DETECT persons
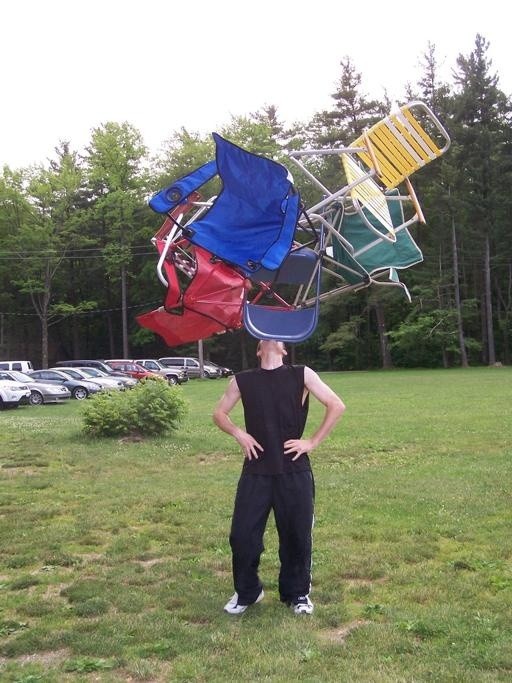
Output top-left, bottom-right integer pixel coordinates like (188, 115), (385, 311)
(211, 334), (346, 618)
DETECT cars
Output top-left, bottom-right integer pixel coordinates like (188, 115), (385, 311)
(0, 356), (232, 411)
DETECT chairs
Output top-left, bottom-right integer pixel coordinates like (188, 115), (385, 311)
(151, 133), (299, 275)
(287, 101), (451, 242)
(138, 191), (246, 348)
(301, 187), (429, 312)
(241, 210), (330, 343)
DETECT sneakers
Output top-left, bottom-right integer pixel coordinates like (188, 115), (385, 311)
(224, 589), (266, 615)
(292, 594), (313, 615)
